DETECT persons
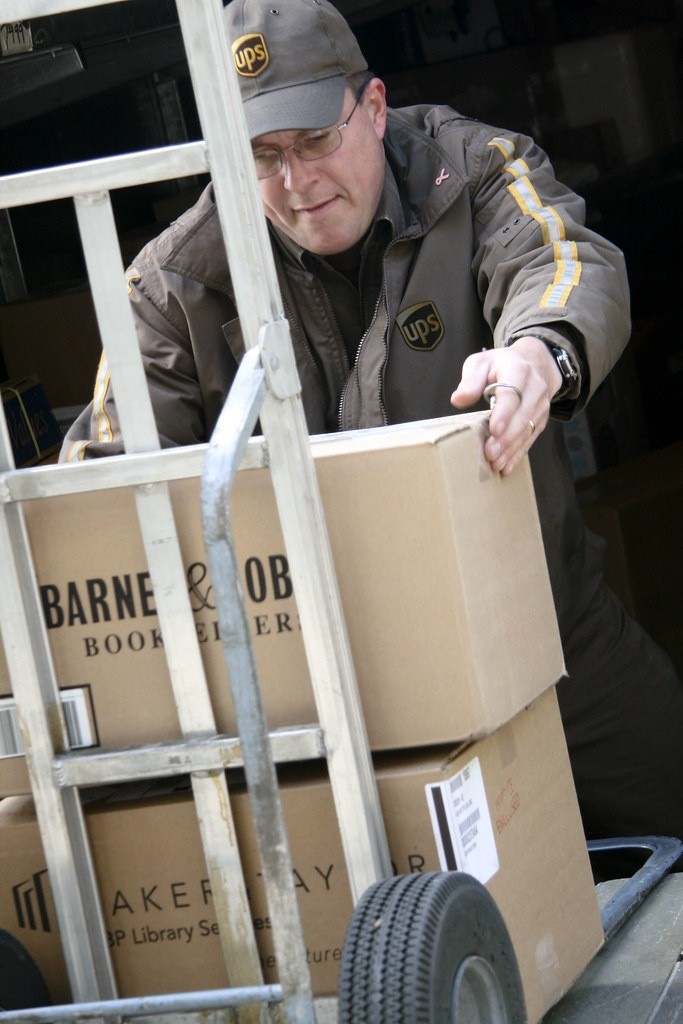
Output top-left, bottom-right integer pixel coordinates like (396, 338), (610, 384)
(60, 0), (683, 844)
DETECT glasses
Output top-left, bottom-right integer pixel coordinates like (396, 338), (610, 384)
(252, 92), (371, 180)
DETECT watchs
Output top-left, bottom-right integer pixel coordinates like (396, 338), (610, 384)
(553, 344), (578, 397)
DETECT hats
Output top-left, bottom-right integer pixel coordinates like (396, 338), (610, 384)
(225, 0), (369, 139)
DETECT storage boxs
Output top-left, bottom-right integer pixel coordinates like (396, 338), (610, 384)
(0, 24), (683, 1024)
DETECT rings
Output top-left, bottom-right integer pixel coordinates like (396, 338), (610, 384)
(530, 420), (536, 430)
